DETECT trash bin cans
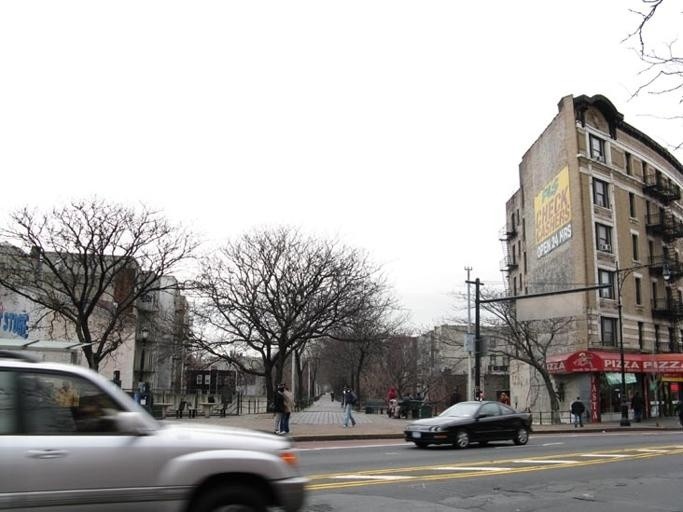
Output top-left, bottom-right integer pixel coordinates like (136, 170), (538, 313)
(410, 400), (433, 419)
(134, 382), (153, 415)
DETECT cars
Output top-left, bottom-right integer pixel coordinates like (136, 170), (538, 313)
(1, 357), (309, 512)
(403, 400), (533, 449)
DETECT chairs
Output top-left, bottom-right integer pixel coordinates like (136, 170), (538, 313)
(170, 399), (200, 418)
(215, 401), (227, 418)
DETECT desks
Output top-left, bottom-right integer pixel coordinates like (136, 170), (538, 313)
(152, 402), (172, 418)
(199, 402), (216, 418)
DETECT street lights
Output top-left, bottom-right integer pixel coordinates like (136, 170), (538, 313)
(138, 327), (150, 382)
(615, 260), (671, 426)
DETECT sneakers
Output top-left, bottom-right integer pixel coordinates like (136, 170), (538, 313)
(275, 428), (286, 434)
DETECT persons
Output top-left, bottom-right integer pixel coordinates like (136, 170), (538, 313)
(630, 391), (644, 422)
(571, 396), (585, 427)
(342, 384), (358, 428)
(499, 392), (510, 406)
(278, 383), (292, 434)
(53, 380), (79, 407)
(331, 391), (335, 401)
(272, 384), (284, 434)
(385, 387), (422, 418)
